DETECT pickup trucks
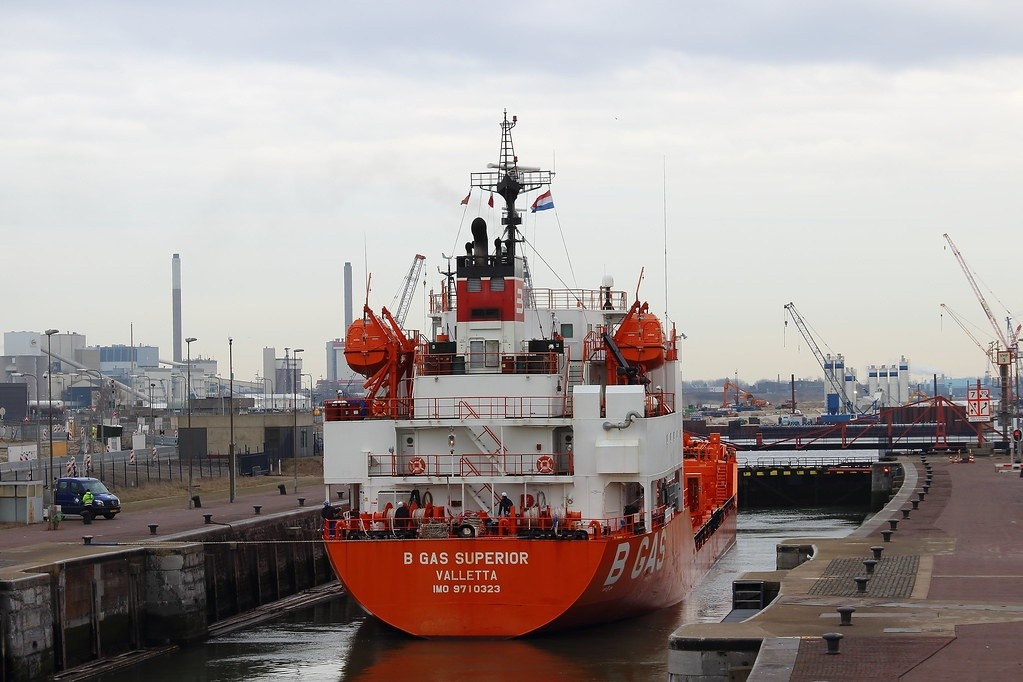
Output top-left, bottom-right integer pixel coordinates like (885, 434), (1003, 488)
(55, 477), (121, 521)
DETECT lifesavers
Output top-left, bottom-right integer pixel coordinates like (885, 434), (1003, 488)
(457, 524), (475, 538)
(537, 456), (553, 473)
(409, 457), (425, 474)
(372, 401), (388, 418)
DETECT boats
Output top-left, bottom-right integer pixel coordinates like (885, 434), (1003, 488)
(613, 313), (665, 372)
(343, 317), (401, 378)
(319, 108), (738, 639)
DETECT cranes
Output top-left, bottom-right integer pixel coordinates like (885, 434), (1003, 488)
(939, 233), (1023, 442)
(783, 302), (880, 424)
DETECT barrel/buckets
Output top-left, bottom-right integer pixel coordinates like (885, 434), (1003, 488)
(516, 355), (557, 373)
(501, 356), (514, 373)
(425, 356), (466, 375)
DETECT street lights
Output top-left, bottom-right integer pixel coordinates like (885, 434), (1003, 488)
(10, 372), (41, 473)
(68, 373), (93, 473)
(51, 375), (67, 422)
(151, 383), (156, 418)
(77, 368), (105, 482)
(294, 349), (305, 495)
(129, 375), (154, 449)
(45, 328), (60, 530)
(265, 379), (274, 414)
(207, 376), (220, 415)
(185, 337), (198, 510)
(227, 337), (235, 504)
(299, 373), (312, 409)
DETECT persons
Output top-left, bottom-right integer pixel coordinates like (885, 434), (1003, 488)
(394, 502), (410, 538)
(321, 500), (342, 538)
(653, 385), (661, 394)
(498, 492), (513, 517)
(82, 489), (95, 518)
(623, 506), (634, 527)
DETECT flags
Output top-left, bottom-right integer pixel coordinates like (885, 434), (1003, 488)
(488, 193), (494, 208)
(461, 190), (471, 204)
(530, 190), (554, 213)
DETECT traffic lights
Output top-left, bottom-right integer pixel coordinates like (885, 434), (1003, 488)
(1013, 430), (1022, 443)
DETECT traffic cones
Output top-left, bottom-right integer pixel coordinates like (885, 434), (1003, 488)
(953, 455), (959, 464)
(948, 455), (953, 464)
(968, 454), (975, 464)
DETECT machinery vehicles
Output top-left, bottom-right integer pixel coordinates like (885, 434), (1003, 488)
(720, 377), (768, 408)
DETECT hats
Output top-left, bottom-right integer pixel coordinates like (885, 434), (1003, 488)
(323, 500), (328, 505)
(502, 492), (507, 496)
(398, 502), (403, 507)
